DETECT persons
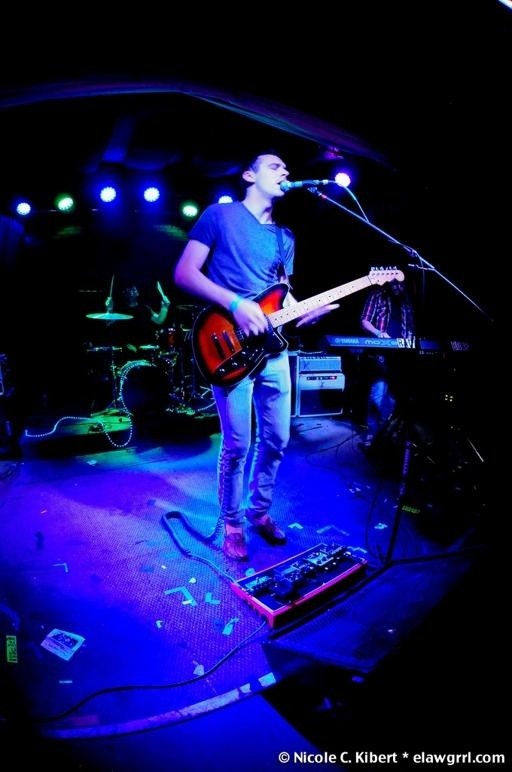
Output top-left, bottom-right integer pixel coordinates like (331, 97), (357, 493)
(105, 285), (171, 344)
(358, 278), (416, 449)
(174, 153), (340, 562)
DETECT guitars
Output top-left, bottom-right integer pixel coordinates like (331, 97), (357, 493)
(192, 266), (404, 387)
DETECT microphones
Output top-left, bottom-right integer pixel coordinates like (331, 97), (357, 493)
(279, 178), (329, 192)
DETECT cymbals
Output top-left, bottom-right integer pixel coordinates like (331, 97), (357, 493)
(86, 313), (134, 321)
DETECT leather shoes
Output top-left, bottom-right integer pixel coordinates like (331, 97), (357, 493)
(220, 523), (247, 563)
(246, 509), (285, 548)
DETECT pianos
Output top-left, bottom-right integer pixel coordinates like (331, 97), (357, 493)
(327, 333), (439, 350)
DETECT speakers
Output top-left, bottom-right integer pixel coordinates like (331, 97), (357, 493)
(262, 550), (481, 731)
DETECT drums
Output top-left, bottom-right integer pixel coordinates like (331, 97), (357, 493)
(158, 328), (180, 356)
(119, 360), (169, 415)
